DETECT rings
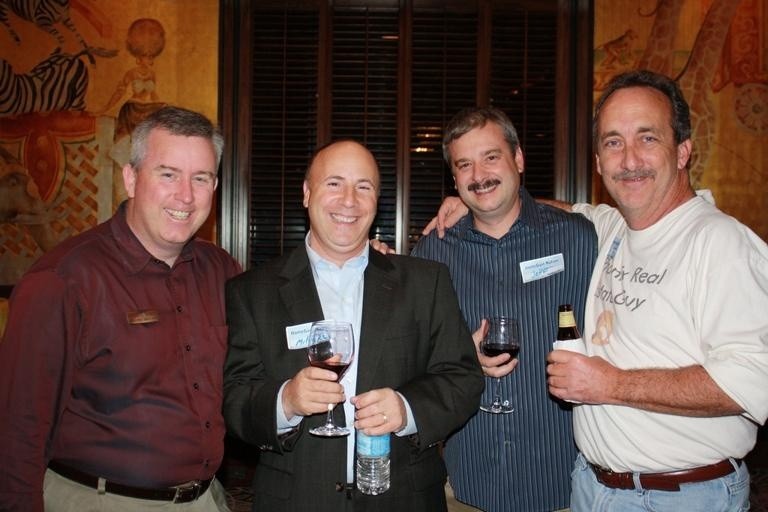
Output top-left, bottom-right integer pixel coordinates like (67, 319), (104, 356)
(381, 411), (389, 425)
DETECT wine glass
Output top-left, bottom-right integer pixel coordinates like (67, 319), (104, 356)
(307, 320), (355, 436)
(477, 316), (520, 414)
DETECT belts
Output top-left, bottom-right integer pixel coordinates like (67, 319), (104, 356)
(46, 458), (216, 505)
(588, 456), (743, 497)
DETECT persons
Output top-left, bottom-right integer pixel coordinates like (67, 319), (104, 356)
(0, 106), (397, 512)
(406, 107), (599, 511)
(223, 138), (486, 512)
(422, 69), (767, 511)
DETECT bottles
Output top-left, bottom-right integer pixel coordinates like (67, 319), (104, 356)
(354, 421), (392, 495)
(552, 304), (591, 404)
